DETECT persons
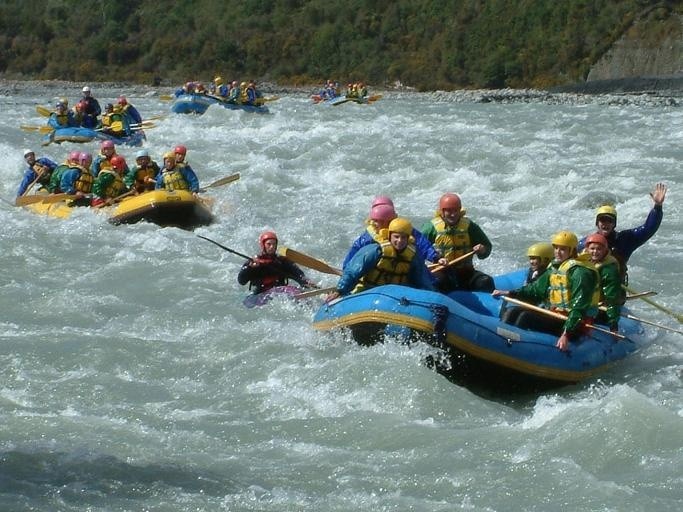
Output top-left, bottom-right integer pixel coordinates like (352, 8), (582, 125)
(16, 140), (199, 210)
(366, 195), (394, 224)
(319, 80), (368, 102)
(46, 85), (142, 139)
(237, 232), (313, 296)
(575, 233), (624, 341)
(524, 242), (554, 308)
(341, 205), (450, 271)
(577, 182), (667, 325)
(491, 231), (601, 352)
(175, 76), (265, 107)
(325, 218), (437, 303)
(418, 192), (495, 295)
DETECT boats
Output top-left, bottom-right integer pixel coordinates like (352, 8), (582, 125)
(312, 265), (650, 384)
(171, 93), (273, 115)
(14, 184), (217, 227)
(46, 124), (146, 146)
(328, 95), (369, 106)
(241, 284), (316, 313)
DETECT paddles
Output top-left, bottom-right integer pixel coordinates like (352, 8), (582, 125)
(197, 235), (321, 292)
(159, 96), (172, 100)
(202, 173), (240, 188)
(42, 195), (81, 204)
(38, 126), (54, 134)
(15, 195), (48, 207)
(254, 98), (266, 103)
(142, 114), (162, 121)
(621, 284), (682, 325)
(37, 107), (51, 117)
(625, 291), (656, 300)
(294, 263), (440, 299)
(93, 122), (153, 132)
(495, 294), (644, 346)
(19, 125), (41, 131)
(285, 248), (363, 286)
(130, 125), (156, 130)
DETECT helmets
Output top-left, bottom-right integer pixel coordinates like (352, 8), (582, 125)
(215, 78), (222, 82)
(59, 97), (68, 103)
(260, 231), (277, 251)
(79, 152), (92, 165)
(596, 205), (617, 226)
(372, 197), (393, 207)
(76, 103), (86, 108)
(440, 194), (461, 217)
(101, 141), (114, 153)
(105, 103), (113, 109)
(249, 83), (255, 88)
(388, 217), (412, 238)
(111, 156), (125, 170)
(552, 230), (578, 256)
(175, 146), (186, 155)
(118, 97), (126, 104)
(527, 243), (554, 265)
(186, 82), (193, 88)
(56, 101), (64, 107)
(371, 205), (395, 220)
(197, 84), (203, 88)
(233, 81), (238, 86)
(82, 86), (91, 92)
(136, 150), (149, 161)
(585, 234), (608, 247)
(163, 151), (176, 165)
(70, 152), (81, 163)
(240, 82), (246, 86)
(24, 149), (34, 159)
